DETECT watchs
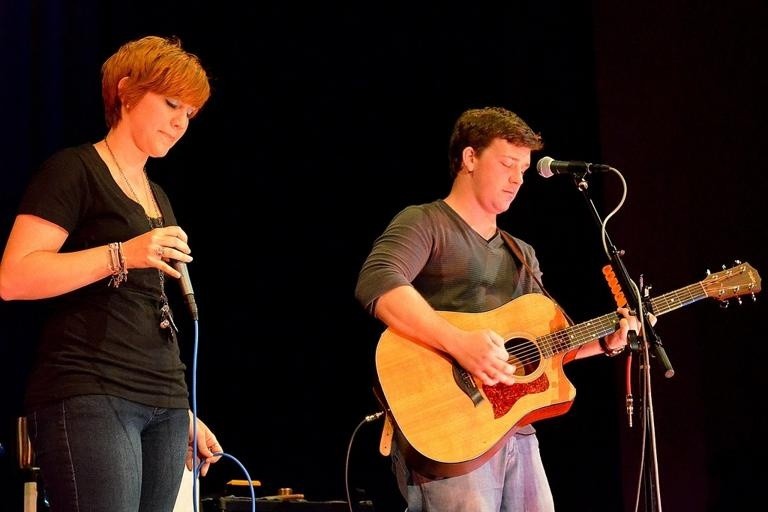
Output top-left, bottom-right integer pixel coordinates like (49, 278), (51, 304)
(599, 336), (626, 357)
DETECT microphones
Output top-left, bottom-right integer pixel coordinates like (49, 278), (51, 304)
(168, 244), (200, 320)
(534, 157), (610, 179)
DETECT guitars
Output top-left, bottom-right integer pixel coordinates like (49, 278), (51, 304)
(371, 260), (761, 480)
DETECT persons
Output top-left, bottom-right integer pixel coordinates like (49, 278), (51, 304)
(354, 108), (658, 512)
(0, 35), (224, 511)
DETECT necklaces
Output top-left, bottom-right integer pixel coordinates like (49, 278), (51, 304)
(104, 135), (165, 294)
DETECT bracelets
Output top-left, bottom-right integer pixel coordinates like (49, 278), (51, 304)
(110, 241), (128, 275)
